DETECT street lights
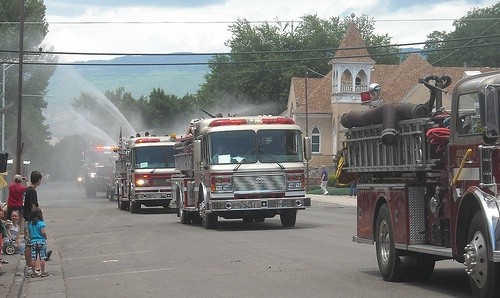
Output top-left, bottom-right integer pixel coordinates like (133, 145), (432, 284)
(1, 60), (46, 149)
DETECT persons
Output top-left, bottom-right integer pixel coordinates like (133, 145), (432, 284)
(23, 171), (42, 278)
(320, 165), (329, 196)
(7, 174), (31, 221)
(10, 210), (26, 254)
(0, 201), (9, 271)
(28, 207), (49, 278)
(350, 182), (356, 197)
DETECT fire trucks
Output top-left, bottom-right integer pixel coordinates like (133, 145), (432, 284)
(340, 69), (500, 298)
(111, 126), (182, 214)
(81, 145), (121, 200)
(167, 110), (312, 229)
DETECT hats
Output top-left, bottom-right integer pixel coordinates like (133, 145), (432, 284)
(14, 174), (23, 182)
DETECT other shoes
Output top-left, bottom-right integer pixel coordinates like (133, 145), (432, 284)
(45, 249), (52, 261)
(25, 266), (33, 277)
(41, 273), (49, 277)
(0, 258), (8, 264)
(31, 272), (41, 277)
